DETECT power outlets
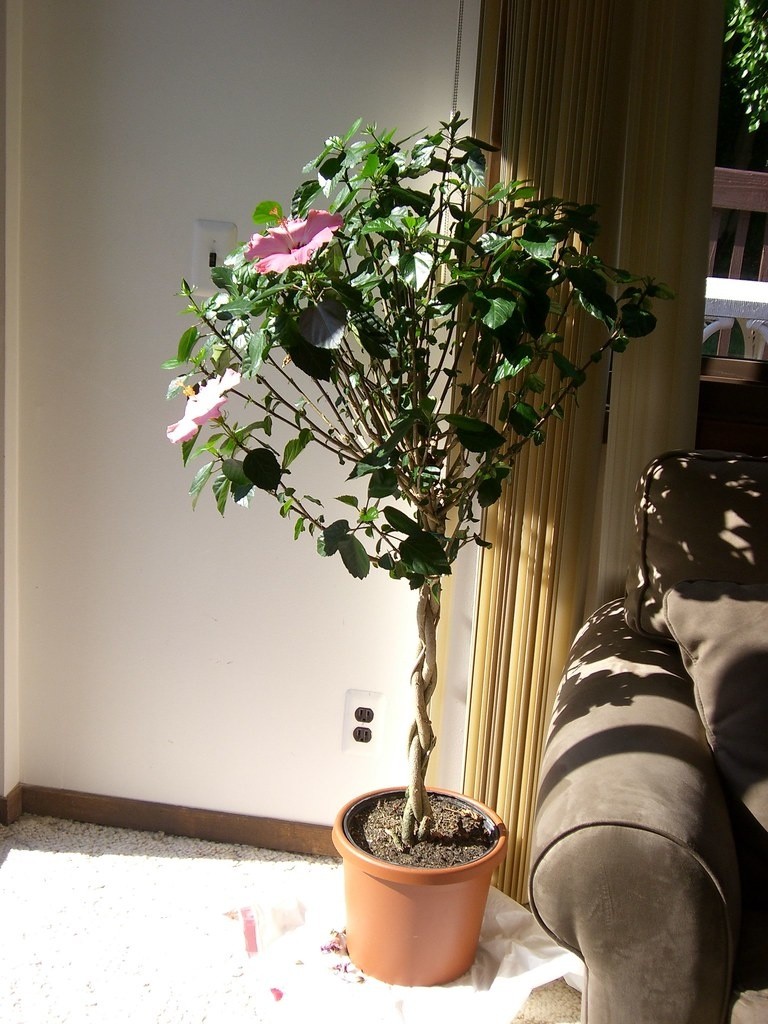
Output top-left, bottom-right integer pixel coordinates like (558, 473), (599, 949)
(342, 689), (383, 760)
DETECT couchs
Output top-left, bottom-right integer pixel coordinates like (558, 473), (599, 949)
(528, 451), (768, 1024)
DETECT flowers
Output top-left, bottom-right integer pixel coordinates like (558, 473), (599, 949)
(160, 107), (678, 845)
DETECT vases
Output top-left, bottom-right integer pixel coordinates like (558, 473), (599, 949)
(332, 786), (507, 988)
